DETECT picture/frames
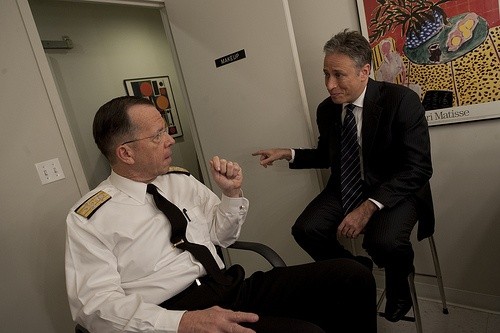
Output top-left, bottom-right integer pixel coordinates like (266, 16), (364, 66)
(123, 75), (183, 139)
(355, 0), (500, 127)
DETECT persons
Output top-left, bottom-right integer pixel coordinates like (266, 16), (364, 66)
(65, 95), (377, 333)
(251, 31), (433, 323)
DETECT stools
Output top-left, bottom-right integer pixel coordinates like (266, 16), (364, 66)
(351, 236), (449, 333)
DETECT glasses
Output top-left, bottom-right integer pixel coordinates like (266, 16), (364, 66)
(122, 127), (171, 144)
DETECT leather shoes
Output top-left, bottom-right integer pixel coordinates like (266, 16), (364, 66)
(385, 267), (415, 322)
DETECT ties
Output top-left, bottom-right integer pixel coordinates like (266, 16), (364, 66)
(340, 104), (360, 217)
(146, 184), (221, 275)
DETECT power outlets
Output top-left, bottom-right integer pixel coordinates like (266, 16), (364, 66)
(35, 158), (66, 185)
